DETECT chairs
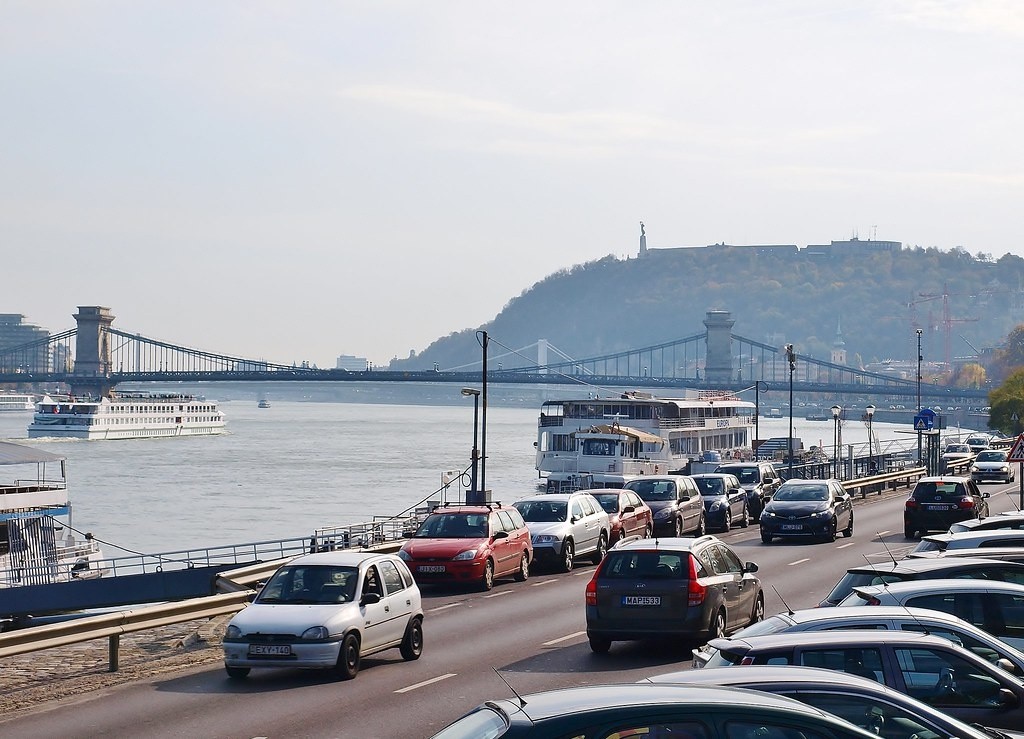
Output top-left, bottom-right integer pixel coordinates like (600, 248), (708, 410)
(961, 448), (967, 452)
(969, 440), (976, 444)
(951, 447), (957, 452)
(981, 454), (989, 459)
(340, 575), (369, 600)
(925, 484), (938, 494)
(445, 517), (469, 536)
(713, 484), (723, 493)
(787, 487), (825, 498)
(296, 570), (332, 597)
(663, 482), (674, 496)
(473, 520), (488, 535)
(696, 480), (708, 495)
(635, 555), (661, 572)
(749, 471), (757, 481)
(844, 649), (878, 683)
(637, 484), (656, 497)
(553, 506), (567, 519)
(953, 484), (966, 495)
(528, 505), (542, 517)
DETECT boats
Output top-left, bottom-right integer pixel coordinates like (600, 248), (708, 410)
(805, 412), (828, 421)
(764, 408), (784, 418)
(26, 385), (230, 441)
(257, 398), (272, 409)
(0, 438), (111, 590)
(533, 386), (759, 495)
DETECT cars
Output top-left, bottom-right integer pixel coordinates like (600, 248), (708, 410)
(633, 663), (1023, 739)
(903, 476), (991, 539)
(395, 501), (534, 591)
(512, 493), (610, 572)
(687, 473), (750, 533)
(962, 437), (991, 455)
(941, 443), (974, 461)
(759, 402), (992, 413)
(758, 478), (854, 544)
(573, 486), (655, 549)
(428, 684), (887, 739)
(692, 509), (1024, 731)
(221, 551), (424, 680)
(970, 449), (1016, 485)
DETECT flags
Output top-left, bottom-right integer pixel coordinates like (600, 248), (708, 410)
(53, 405), (60, 414)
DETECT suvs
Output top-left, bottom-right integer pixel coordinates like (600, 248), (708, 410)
(707, 460), (783, 525)
(621, 474), (707, 538)
(583, 533), (766, 655)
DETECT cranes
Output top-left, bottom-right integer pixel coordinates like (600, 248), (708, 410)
(901, 281), (1016, 386)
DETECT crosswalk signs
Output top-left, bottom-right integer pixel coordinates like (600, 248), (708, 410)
(1004, 434), (1024, 463)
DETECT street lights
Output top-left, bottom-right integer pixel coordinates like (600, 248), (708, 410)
(459, 386), (482, 505)
(829, 404), (841, 481)
(865, 405), (875, 474)
